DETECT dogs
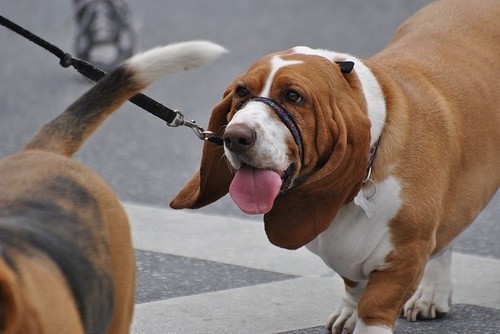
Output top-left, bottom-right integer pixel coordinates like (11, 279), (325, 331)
(169, 1), (498, 334)
(1, 40), (231, 334)
